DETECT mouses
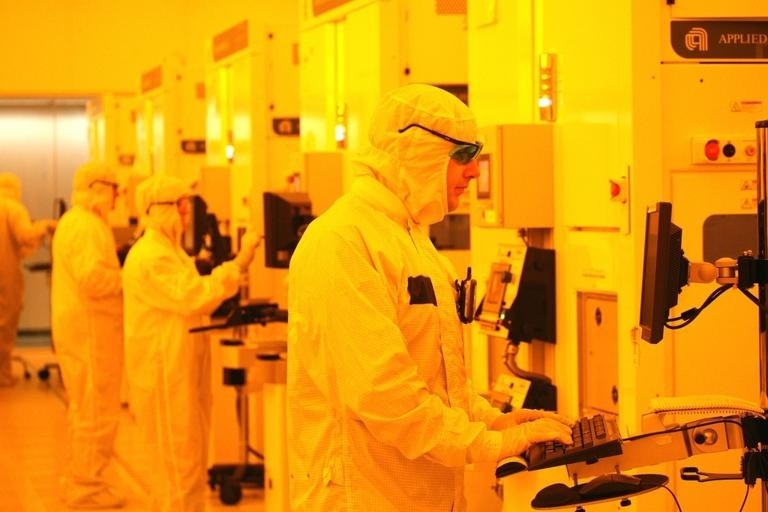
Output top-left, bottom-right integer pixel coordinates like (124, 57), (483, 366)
(578, 473), (641, 499)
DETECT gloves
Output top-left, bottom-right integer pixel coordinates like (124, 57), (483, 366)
(465, 405), (577, 465)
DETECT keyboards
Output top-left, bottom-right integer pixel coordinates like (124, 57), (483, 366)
(528, 414), (623, 469)
(239, 304), (278, 316)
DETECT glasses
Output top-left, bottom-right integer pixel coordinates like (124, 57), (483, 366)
(397, 122), (485, 165)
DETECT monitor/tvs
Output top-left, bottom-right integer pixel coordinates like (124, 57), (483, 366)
(639, 202), (682, 344)
(264, 190), (312, 269)
(181, 195), (208, 255)
(53, 198), (66, 220)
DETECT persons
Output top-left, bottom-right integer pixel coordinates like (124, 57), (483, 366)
(50, 160), (126, 510)
(121, 172), (261, 512)
(0, 170), (58, 387)
(283, 80), (575, 512)
